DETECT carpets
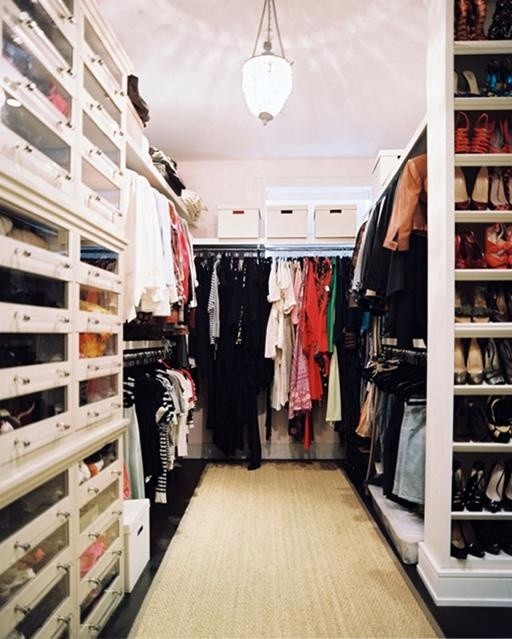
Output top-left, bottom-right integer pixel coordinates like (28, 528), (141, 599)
(127, 459), (446, 638)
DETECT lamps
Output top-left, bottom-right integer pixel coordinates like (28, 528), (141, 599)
(238, 1), (293, 125)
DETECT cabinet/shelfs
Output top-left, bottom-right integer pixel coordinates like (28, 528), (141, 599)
(2, 1), (143, 638)
(413, 0), (512, 613)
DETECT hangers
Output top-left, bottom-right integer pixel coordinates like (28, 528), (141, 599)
(365, 343), (429, 397)
(123, 348), (165, 367)
(195, 247), (358, 273)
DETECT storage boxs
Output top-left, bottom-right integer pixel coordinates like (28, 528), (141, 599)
(267, 205), (309, 242)
(312, 204), (358, 239)
(370, 148), (405, 186)
(122, 499), (152, 593)
(215, 203), (262, 240)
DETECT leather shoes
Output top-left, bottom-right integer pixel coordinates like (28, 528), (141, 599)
(448, 0), (511, 562)
